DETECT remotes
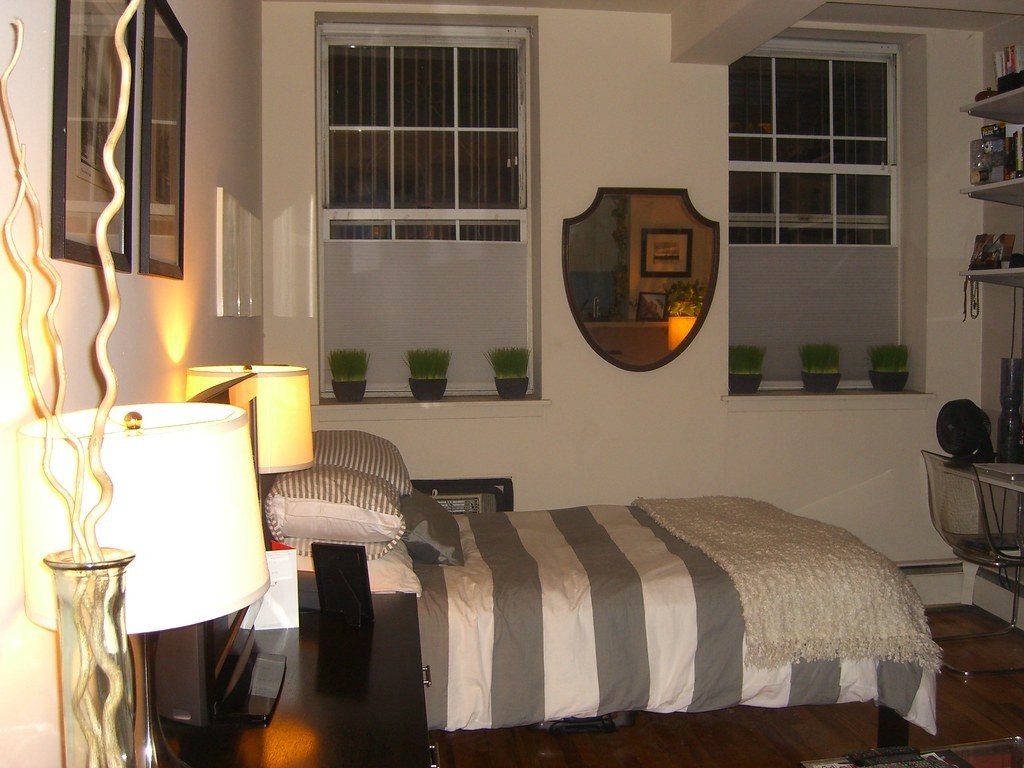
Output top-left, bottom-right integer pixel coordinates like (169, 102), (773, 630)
(237, 652), (288, 721)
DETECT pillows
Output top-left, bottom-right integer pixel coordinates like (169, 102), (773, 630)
(265, 464), (406, 561)
(312, 430), (413, 495)
(400, 489), (464, 567)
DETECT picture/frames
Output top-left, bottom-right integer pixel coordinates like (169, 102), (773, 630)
(51, 0), (137, 274)
(139, 0), (188, 281)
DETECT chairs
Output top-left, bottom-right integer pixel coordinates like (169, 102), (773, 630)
(921, 450), (1024, 676)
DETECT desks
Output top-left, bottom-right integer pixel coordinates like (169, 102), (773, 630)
(943, 454), (1024, 492)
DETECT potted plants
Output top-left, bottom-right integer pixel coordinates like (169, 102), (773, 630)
(798, 342), (841, 392)
(401, 349), (451, 400)
(729, 345), (766, 393)
(328, 348), (370, 402)
(482, 346), (531, 399)
(867, 344), (910, 391)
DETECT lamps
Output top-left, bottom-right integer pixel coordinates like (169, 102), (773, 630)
(187, 364), (314, 474)
(20, 402), (271, 768)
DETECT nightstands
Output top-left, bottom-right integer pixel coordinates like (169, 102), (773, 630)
(161, 593), (440, 768)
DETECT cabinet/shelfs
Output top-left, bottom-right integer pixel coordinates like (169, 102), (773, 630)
(959, 88), (1024, 288)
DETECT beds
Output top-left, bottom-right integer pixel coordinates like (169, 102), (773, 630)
(186, 373), (943, 748)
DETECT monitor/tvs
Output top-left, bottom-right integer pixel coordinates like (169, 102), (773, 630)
(148, 596), (264, 728)
